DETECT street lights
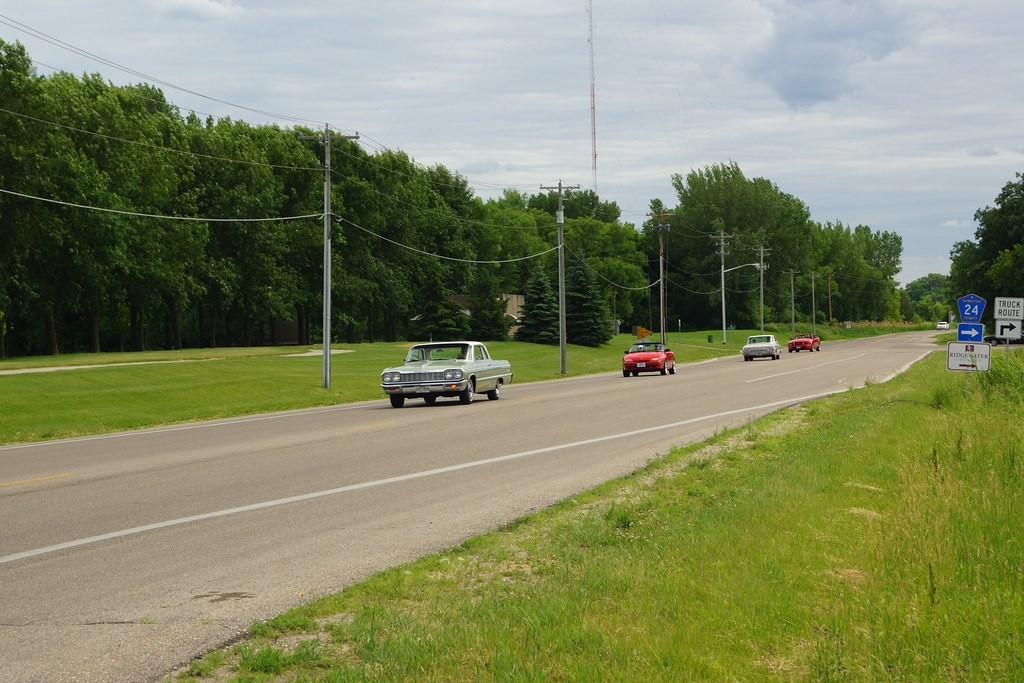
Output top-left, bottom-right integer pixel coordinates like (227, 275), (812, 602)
(721, 262), (760, 343)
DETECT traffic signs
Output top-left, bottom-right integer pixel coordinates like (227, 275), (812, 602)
(947, 341), (992, 373)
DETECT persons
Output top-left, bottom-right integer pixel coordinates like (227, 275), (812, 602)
(653, 345), (663, 352)
(638, 345), (646, 352)
(456, 346), (479, 360)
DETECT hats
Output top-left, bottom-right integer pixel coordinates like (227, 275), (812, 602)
(638, 345), (644, 349)
(655, 344), (659, 347)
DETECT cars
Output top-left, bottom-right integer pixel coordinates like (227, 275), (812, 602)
(742, 335), (782, 361)
(936, 322), (950, 330)
(380, 341), (514, 408)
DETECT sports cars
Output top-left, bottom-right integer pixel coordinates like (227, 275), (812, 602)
(788, 333), (821, 353)
(622, 342), (675, 377)
